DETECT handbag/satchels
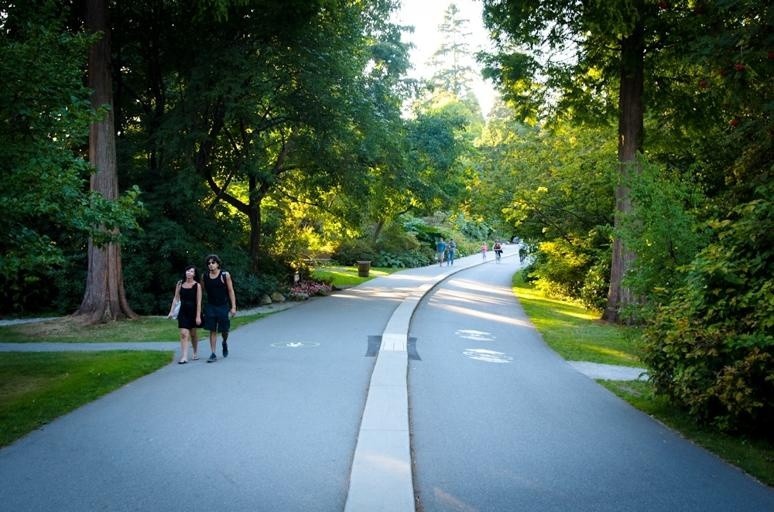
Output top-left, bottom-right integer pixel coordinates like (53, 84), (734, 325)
(166, 280), (181, 320)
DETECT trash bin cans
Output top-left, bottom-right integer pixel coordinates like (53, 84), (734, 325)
(357, 261), (371, 277)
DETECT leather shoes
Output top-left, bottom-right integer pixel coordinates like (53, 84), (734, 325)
(178, 359), (187, 363)
(191, 352), (198, 360)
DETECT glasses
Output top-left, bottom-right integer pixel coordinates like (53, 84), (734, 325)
(207, 262), (214, 265)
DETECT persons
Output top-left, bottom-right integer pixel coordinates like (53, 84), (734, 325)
(446, 238), (456, 266)
(436, 237), (446, 267)
(491, 239), (503, 258)
(481, 240), (488, 261)
(165, 264), (203, 364)
(197, 253), (237, 363)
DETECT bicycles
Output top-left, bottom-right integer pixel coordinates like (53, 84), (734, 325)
(493, 248), (503, 264)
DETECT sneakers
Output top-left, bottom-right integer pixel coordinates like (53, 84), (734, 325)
(222, 341), (227, 357)
(207, 354), (216, 362)
